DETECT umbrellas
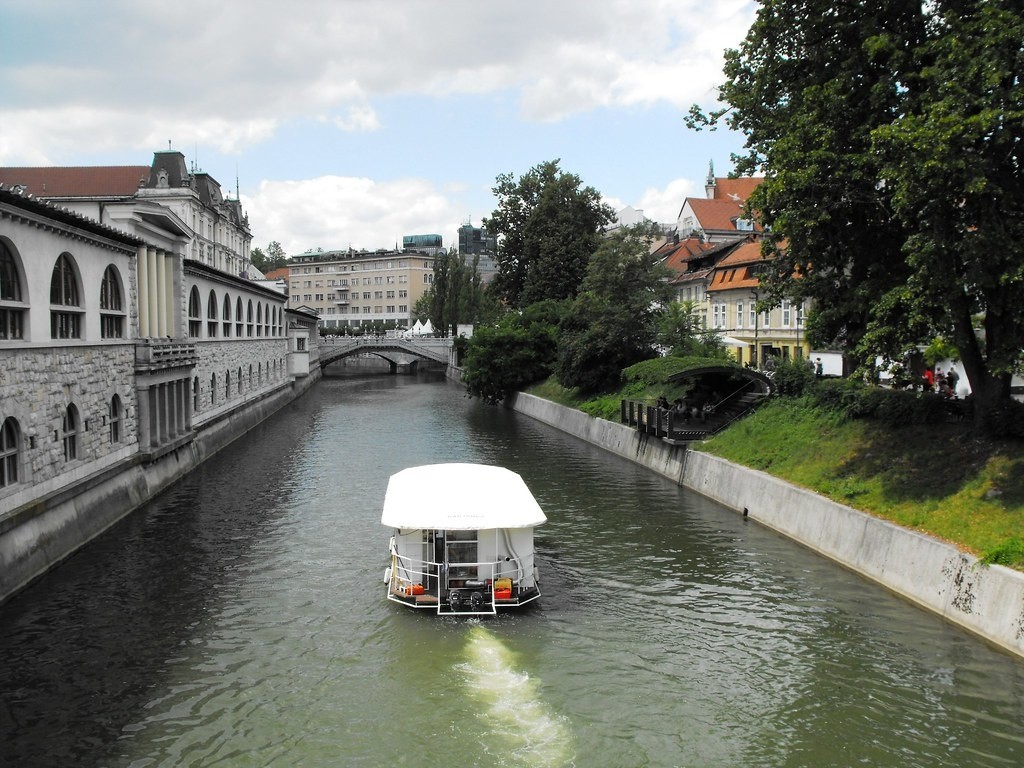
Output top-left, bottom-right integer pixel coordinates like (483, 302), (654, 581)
(693, 333), (749, 351)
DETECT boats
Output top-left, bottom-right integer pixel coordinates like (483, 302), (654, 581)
(380, 463), (547, 615)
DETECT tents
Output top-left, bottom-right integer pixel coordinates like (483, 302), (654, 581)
(405, 318), (440, 335)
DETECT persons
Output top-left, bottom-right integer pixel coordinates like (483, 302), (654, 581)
(937, 373), (951, 400)
(382, 335), (384, 343)
(921, 367), (935, 393)
(331, 334), (335, 344)
(815, 358), (823, 375)
(325, 334), (328, 343)
(936, 368), (943, 376)
(806, 356), (815, 370)
(661, 347), (667, 357)
(945, 372), (956, 397)
(951, 367), (960, 395)
(745, 360), (755, 370)
(363, 332), (379, 344)
(355, 335), (359, 346)
